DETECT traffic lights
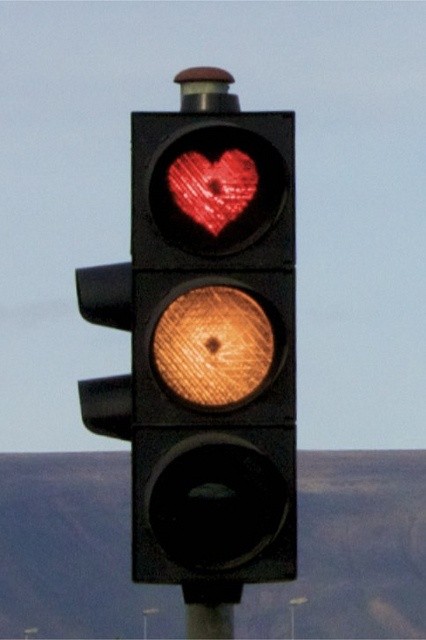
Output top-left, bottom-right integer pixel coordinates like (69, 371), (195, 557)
(73, 243), (130, 441)
(128, 93), (298, 609)
